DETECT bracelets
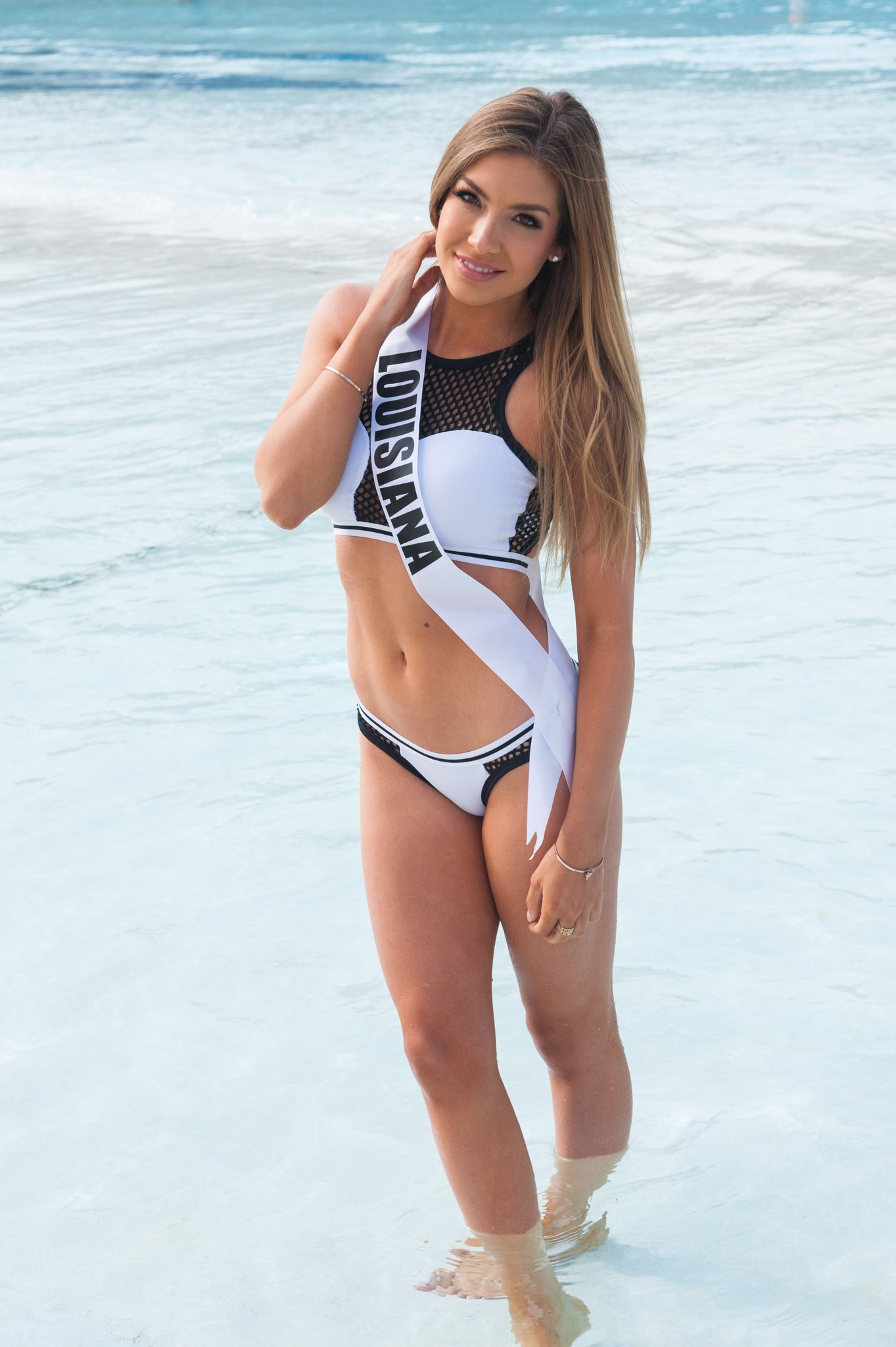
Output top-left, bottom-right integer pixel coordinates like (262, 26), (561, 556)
(322, 367), (367, 403)
(554, 841), (604, 880)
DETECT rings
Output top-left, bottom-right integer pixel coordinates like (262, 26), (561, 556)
(557, 923), (574, 935)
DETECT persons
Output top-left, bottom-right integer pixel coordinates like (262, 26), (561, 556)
(254, 89), (650, 1347)
